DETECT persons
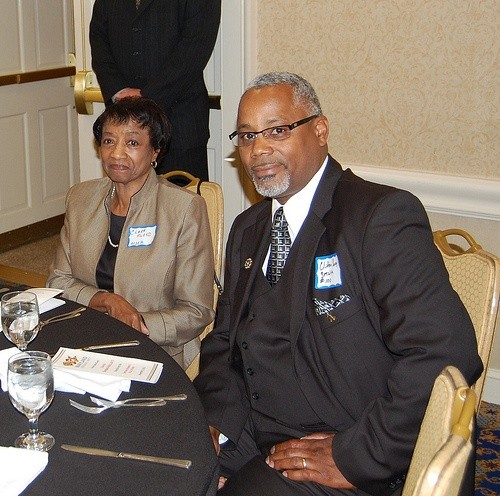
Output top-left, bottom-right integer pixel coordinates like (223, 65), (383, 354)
(88, 0), (221, 186)
(193, 71), (480, 496)
(46, 95), (215, 371)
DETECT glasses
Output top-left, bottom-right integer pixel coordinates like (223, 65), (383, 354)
(228, 114), (320, 148)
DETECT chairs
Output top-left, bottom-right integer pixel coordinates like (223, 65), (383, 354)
(403, 366), (481, 495)
(159, 171), (225, 380)
(433, 227), (499, 411)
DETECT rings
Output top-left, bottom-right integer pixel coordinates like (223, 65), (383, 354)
(303, 458), (306, 468)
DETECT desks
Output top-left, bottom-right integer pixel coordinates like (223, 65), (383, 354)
(0, 279), (220, 496)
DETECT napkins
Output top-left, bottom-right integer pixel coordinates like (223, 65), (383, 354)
(51, 367), (134, 401)
(0, 344), (22, 392)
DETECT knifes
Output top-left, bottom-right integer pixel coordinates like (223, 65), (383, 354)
(60, 444), (192, 470)
(45, 340), (140, 356)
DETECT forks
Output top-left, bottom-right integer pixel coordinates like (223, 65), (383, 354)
(69, 398), (166, 414)
(90, 394), (187, 406)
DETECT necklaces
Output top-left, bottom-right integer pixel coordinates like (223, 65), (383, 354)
(108, 186), (119, 248)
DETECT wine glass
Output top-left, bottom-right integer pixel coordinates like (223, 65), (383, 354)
(0, 291), (39, 352)
(7, 351), (55, 451)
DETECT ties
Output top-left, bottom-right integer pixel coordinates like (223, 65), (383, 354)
(264, 208), (292, 290)
